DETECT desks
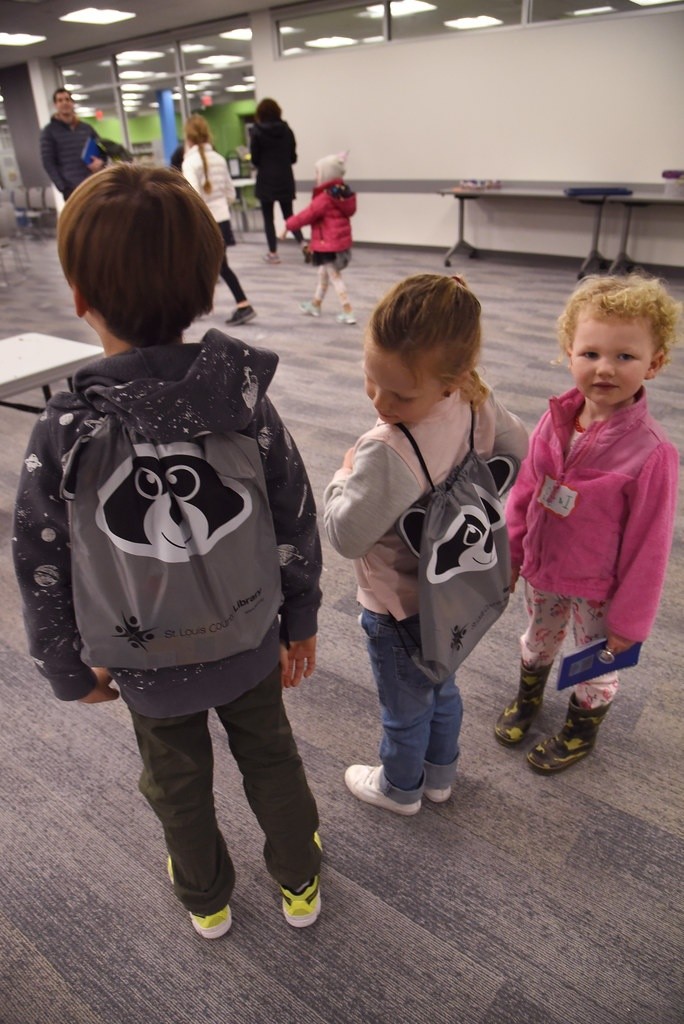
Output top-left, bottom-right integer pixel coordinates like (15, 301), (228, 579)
(440, 186), (684, 280)
(0, 332), (104, 416)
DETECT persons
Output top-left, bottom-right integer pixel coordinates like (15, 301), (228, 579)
(12, 160), (323, 939)
(181, 113), (255, 327)
(170, 143), (185, 173)
(322, 273), (529, 815)
(495, 273), (678, 774)
(249, 99), (308, 264)
(40, 88), (112, 203)
(281, 150), (357, 325)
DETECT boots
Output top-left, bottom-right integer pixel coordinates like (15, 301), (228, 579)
(494, 659), (555, 746)
(526, 691), (614, 773)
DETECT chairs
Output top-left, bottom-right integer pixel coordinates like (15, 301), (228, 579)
(0, 186), (56, 284)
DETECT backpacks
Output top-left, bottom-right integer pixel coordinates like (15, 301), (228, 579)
(49, 401), (285, 667)
(391, 400), (510, 682)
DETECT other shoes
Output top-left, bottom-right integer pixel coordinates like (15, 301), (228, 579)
(263, 253), (280, 263)
(226, 306), (255, 326)
(302, 244), (312, 263)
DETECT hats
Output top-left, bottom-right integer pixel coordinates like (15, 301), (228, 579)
(316, 148), (349, 184)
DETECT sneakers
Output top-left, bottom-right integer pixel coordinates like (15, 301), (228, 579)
(299, 301), (320, 317)
(281, 829), (322, 927)
(337, 313), (356, 324)
(166, 854), (231, 939)
(424, 781), (451, 803)
(344, 764), (421, 816)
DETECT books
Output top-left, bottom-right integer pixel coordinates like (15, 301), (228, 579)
(557, 636), (642, 690)
(80, 136), (112, 173)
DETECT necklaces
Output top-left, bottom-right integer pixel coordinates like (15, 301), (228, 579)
(575, 409), (584, 433)
